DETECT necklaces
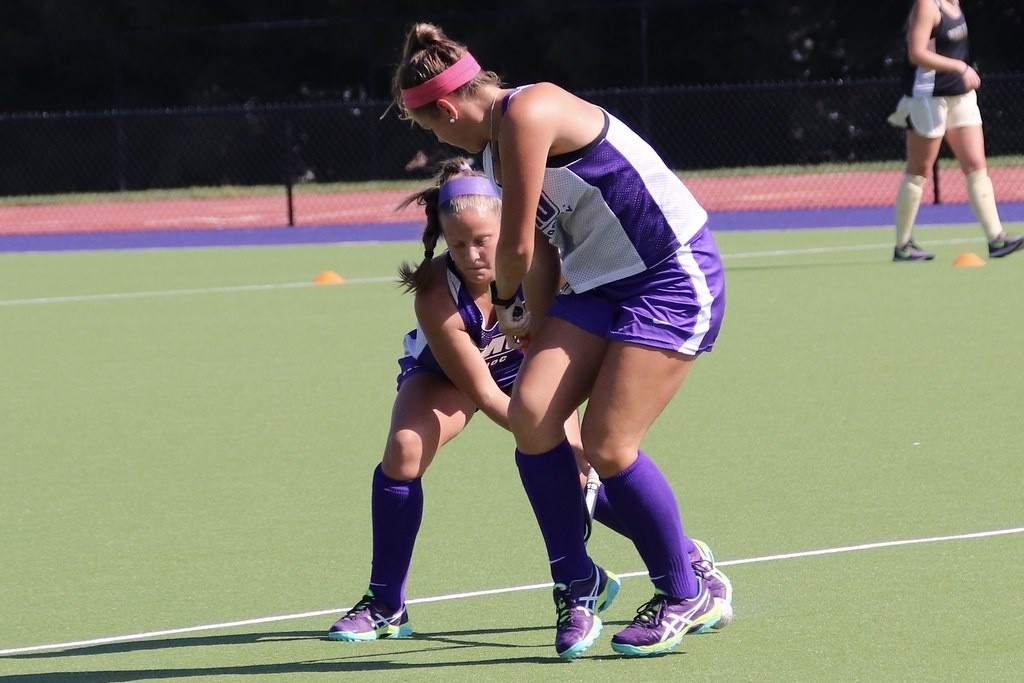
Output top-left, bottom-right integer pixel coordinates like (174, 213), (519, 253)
(489, 88), (501, 155)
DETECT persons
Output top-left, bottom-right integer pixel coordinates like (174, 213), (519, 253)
(888, 0), (1024, 262)
(327, 159), (621, 659)
(394, 23), (727, 657)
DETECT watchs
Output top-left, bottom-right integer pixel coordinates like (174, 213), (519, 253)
(488, 281), (516, 309)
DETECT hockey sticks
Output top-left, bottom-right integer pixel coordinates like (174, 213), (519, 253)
(512, 303), (600, 546)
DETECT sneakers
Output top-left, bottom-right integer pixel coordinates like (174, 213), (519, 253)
(610, 576), (720, 655)
(329, 589), (412, 641)
(686, 539), (733, 604)
(988, 231), (1024, 256)
(893, 240), (935, 262)
(552, 556), (620, 661)
